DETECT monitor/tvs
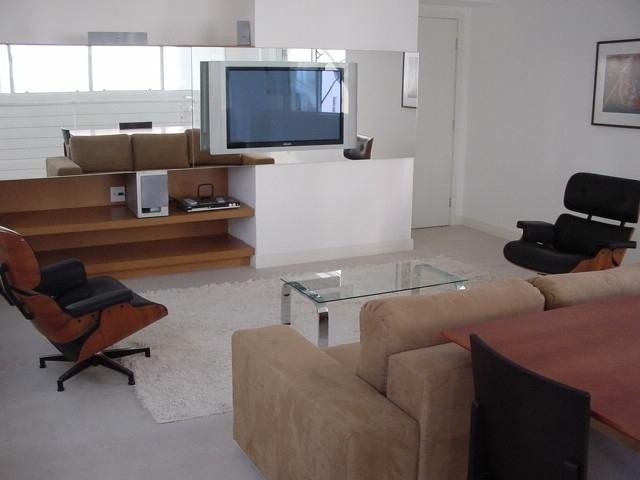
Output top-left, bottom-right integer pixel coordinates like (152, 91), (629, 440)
(200, 62), (210, 151)
(208, 61), (357, 155)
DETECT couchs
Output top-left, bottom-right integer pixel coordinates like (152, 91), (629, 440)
(44, 128), (274, 176)
(230, 259), (640, 477)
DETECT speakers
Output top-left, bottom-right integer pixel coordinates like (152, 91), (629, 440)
(237, 21), (250, 46)
(88, 31), (147, 46)
(126, 169), (169, 219)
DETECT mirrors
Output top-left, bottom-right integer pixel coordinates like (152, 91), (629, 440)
(0, 40), (419, 182)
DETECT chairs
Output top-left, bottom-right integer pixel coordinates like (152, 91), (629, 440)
(0, 224), (169, 392)
(501, 172), (640, 274)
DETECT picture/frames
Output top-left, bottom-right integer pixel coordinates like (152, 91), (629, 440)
(592, 35), (640, 129)
(400, 52), (418, 109)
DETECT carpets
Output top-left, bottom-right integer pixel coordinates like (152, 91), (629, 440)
(121, 256), (493, 426)
